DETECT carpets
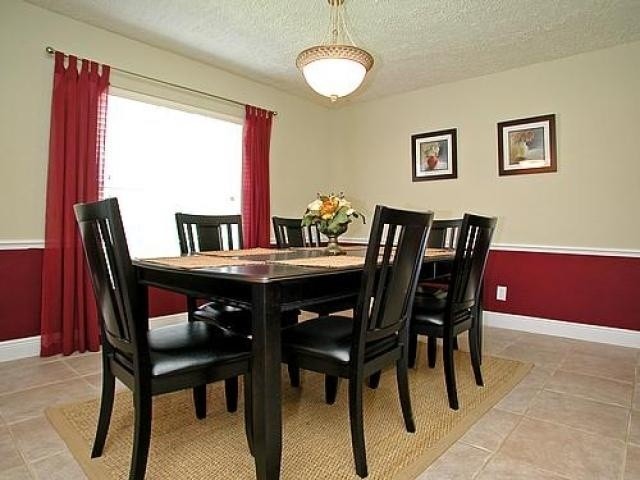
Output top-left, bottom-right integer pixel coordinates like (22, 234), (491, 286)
(44, 339), (534, 480)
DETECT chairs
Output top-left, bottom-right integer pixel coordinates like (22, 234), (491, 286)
(75, 196), (258, 479)
(169, 208), (256, 336)
(271, 214), (368, 326)
(265, 203), (435, 477)
(405, 210), (498, 411)
(405, 214), (469, 369)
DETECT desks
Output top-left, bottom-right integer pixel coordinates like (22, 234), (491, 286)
(128, 237), (479, 478)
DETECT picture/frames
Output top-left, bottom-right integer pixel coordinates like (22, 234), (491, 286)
(498, 114), (557, 176)
(411, 128), (457, 182)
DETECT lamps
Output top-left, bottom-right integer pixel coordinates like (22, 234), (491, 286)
(296, 0), (374, 103)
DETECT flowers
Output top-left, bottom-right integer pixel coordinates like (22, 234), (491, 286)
(300, 192), (367, 234)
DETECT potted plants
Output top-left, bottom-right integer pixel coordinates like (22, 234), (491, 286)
(320, 235), (347, 256)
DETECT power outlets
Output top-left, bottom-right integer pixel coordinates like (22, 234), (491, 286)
(496, 285), (507, 301)
